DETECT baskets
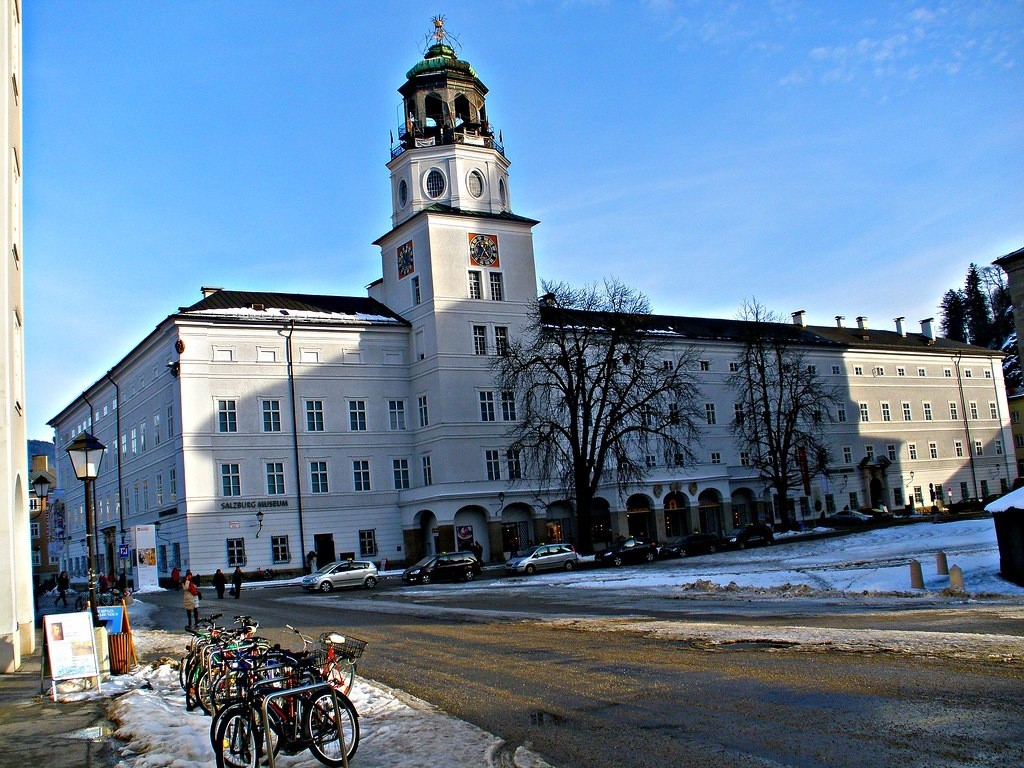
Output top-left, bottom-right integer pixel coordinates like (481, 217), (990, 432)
(319, 631), (368, 658)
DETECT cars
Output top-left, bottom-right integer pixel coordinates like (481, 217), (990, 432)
(986, 494), (1006, 505)
(658, 533), (721, 559)
(401, 551), (481, 584)
(504, 543), (577, 575)
(952, 497), (986, 511)
(301, 561), (379, 593)
(830, 510), (873, 523)
(863, 509), (894, 517)
(722, 525), (774, 550)
(594, 538), (658, 567)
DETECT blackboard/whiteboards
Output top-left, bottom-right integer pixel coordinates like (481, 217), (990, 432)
(380, 557), (389, 571)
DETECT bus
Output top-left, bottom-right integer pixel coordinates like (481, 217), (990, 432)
(1013, 476), (1024, 491)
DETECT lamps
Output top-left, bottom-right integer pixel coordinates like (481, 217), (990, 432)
(80, 539), (88, 554)
(840, 474), (848, 493)
(906, 471), (914, 487)
(495, 492), (505, 516)
(30, 473), (51, 520)
(993, 464), (1000, 480)
(256, 511), (265, 539)
(154, 521), (170, 545)
(120, 530), (132, 541)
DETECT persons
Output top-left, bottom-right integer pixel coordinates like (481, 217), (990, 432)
(820, 511), (825, 519)
(232, 566), (245, 600)
(97, 572), (111, 606)
(509, 537), (520, 560)
(212, 568), (227, 600)
(54, 570), (71, 609)
(170, 567), (179, 591)
(182, 569), (201, 629)
(469, 540), (483, 567)
(844, 505), (849, 510)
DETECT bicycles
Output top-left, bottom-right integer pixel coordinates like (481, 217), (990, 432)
(180, 613), (368, 768)
(75, 589), (91, 611)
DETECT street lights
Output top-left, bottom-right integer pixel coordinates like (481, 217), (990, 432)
(64, 430), (107, 627)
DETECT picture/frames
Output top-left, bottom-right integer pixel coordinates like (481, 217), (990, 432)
(469, 235), (499, 266)
(398, 244), (414, 276)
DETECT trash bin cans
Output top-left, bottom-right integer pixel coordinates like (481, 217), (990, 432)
(340, 552), (355, 561)
(108, 632), (132, 676)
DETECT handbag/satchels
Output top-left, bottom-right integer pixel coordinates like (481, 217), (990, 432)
(188, 582), (198, 596)
(57, 586), (63, 592)
(230, 588), (236, 596)
(179, 578), (181, 584)
